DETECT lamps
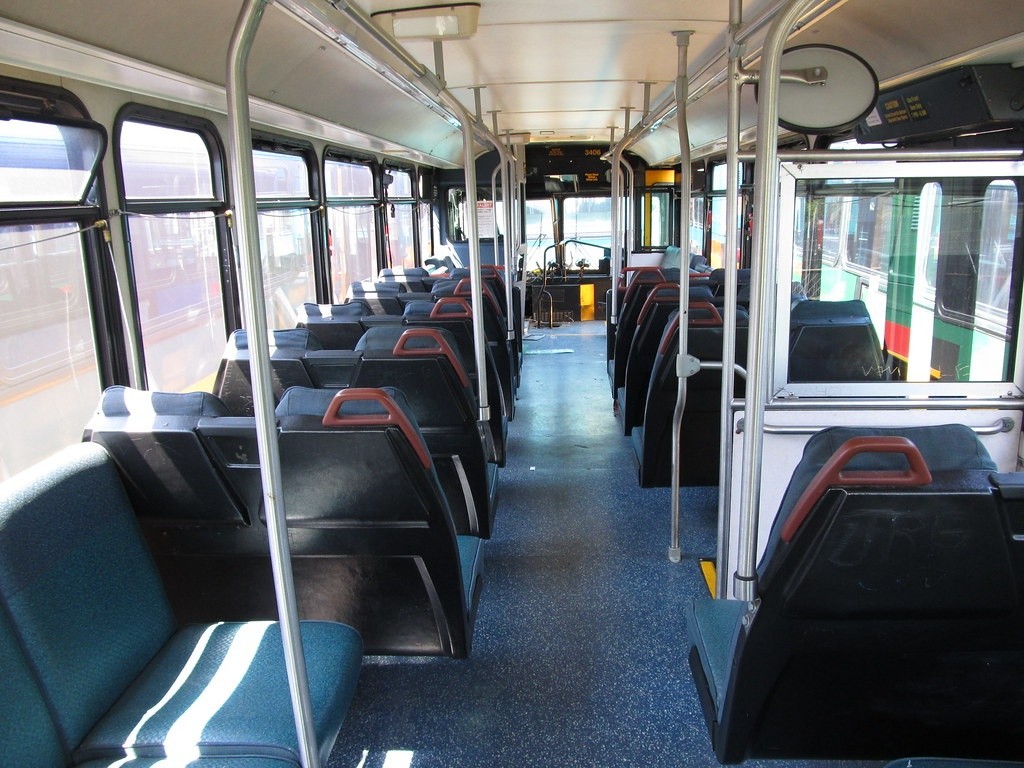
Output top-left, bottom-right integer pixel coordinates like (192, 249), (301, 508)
(370, 1), (481, 40)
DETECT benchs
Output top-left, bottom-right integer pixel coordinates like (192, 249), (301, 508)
(0, 237), (524, 768)
(604, 267), (1024, 768)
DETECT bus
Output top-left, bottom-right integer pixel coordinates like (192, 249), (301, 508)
(689, 138), (1024, 392)
(0, 134), (439, 484)
